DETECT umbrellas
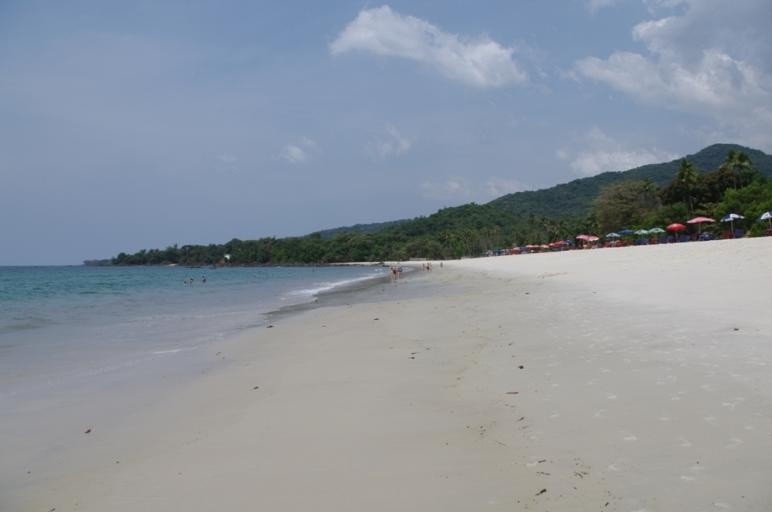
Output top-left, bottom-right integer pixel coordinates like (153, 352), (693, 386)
(512, 221), (688, 253)
(686, 216), (717, 234)
(757, 211), (772, 228)
(720, 213), (746, 239)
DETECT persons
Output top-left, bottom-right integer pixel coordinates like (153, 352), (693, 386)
(390, 264), (402, 281)
(422, 262), (433, 273)
